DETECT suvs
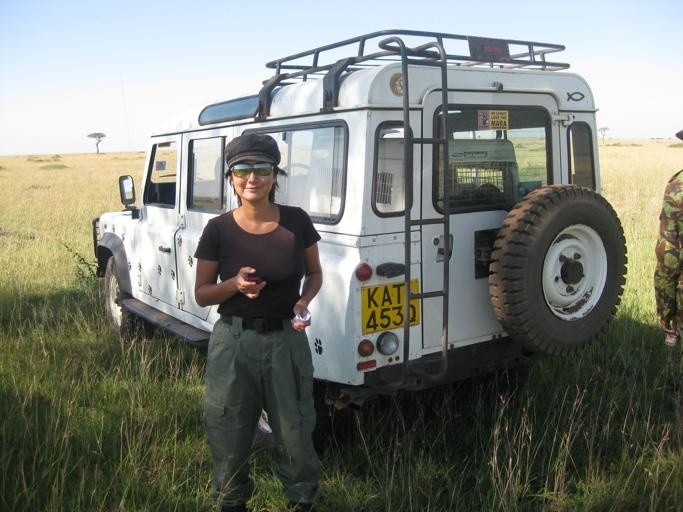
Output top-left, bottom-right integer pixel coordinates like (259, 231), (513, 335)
(87, 26), (635, 417)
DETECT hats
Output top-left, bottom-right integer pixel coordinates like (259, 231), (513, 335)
(225, 133), (281, 166)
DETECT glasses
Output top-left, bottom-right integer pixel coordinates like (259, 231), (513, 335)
(230, 163), (274, 178)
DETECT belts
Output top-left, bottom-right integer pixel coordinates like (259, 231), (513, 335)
(220, 314), (291, 334)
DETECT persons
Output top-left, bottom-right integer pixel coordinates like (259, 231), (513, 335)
(193, 131), (327, 511)
(651, 128), (683, 352)
